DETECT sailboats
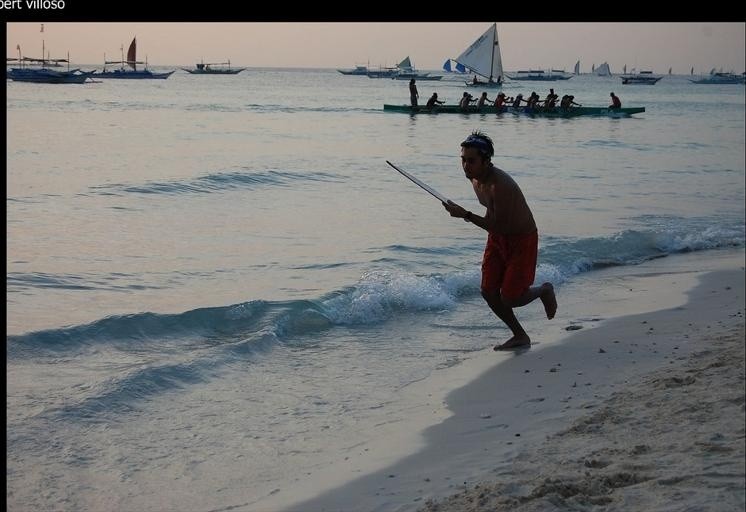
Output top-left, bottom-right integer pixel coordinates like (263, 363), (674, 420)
(335, 22), (505, 88)
(574, 52), (745, 86)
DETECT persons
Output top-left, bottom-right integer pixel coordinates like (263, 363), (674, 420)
(608, 92), (622, 110)
(497, 76), (502, 83)
(459, 89), (584, 109)
(473, 75), (478, 83)
(426, 92), (446, 106)
(410, 78), (420, 106)
(442, 132), (559, 352)
(489, 77), (494, 82)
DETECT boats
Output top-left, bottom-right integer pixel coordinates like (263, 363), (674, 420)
(7, 24), (249, 86)
(383, 104), (647, 119)
(504, 66), (574, 81)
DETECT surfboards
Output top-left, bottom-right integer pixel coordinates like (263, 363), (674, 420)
(384, 158), (450, 204)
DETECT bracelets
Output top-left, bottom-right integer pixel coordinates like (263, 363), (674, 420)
(462, 211), (474, 221)
